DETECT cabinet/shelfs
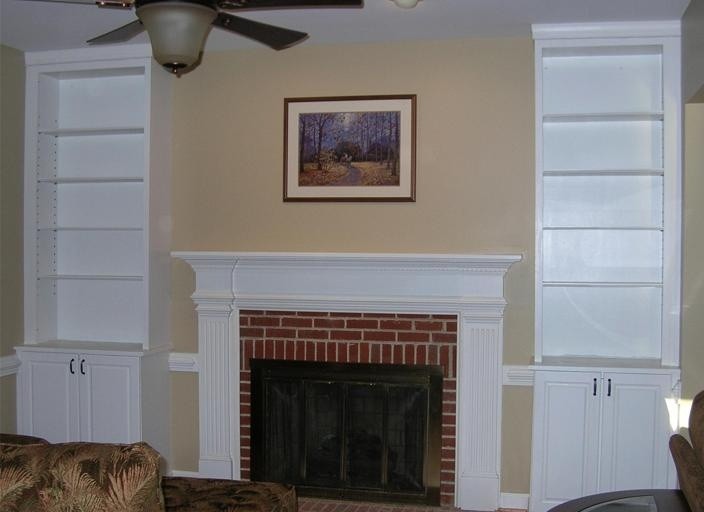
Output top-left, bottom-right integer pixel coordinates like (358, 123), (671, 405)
(530, 19), (682, 512)
(9, 53), (173, 459)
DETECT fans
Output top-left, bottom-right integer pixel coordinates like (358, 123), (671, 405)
(18, 0), (364, 50)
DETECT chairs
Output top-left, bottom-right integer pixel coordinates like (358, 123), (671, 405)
(669, 389), (704, 512)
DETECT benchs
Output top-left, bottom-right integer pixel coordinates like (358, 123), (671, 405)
(0, 432), (298, 512)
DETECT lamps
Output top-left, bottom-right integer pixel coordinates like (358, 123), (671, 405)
(663, 397), (694, 432)
(394, 0), (417, 9)
(135, 3), (216, 76)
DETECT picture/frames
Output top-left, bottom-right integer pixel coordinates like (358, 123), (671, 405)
(281, 94), (417, 202)
